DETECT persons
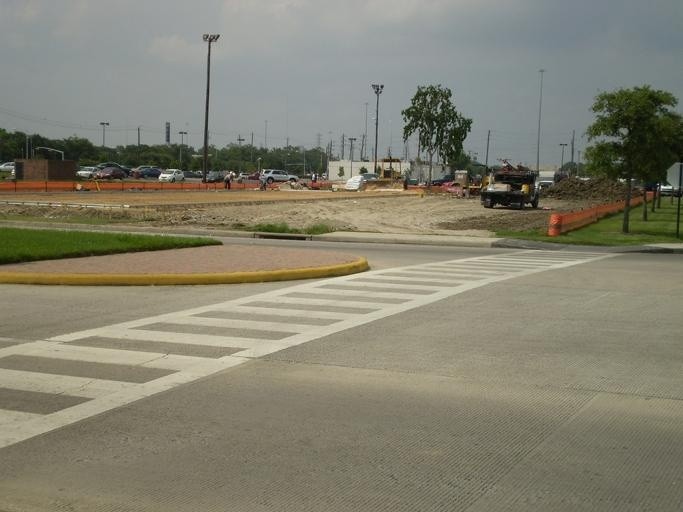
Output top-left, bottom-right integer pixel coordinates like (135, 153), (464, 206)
(401, 174), (407, 190)
(223, 171), (317, 191)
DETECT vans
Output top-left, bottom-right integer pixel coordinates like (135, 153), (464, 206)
(538, 181), (553, 191)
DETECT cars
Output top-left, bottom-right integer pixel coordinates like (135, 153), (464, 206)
(0, 161), (14, 172)
(432, 174), (454, 186)
(656, 181), (678, 197)
(75, 163), (302, 183)
(11, 167), (15, 175)
(361, 172), (379, 184)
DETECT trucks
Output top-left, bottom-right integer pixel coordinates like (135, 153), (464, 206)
(393, 175), (418, 186)
(319, 172), (327, 180)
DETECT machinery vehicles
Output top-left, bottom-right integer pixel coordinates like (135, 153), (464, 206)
(478, 160), (540, 211)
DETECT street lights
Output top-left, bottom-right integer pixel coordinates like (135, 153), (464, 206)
(98, 122), (109, 146)
(10, 126), (36, 160)
(558, 143), (568, 169)
(370, 82), (386, 173)
(535, 70), (544, 178)
(236, 137), (245, 146)
(34, 147), (64, 160)
(177, 131), (187, 144)
(347, 136), (356, 177)
(199, 32), (221, 182)
